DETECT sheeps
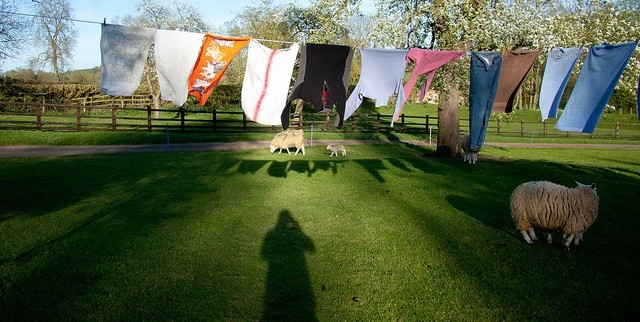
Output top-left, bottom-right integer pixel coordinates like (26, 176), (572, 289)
(459, 134), (482, 165)
(509, 180), (600, 249)
(326, 144), (346, 158)
(270, 129), (305, 156)
